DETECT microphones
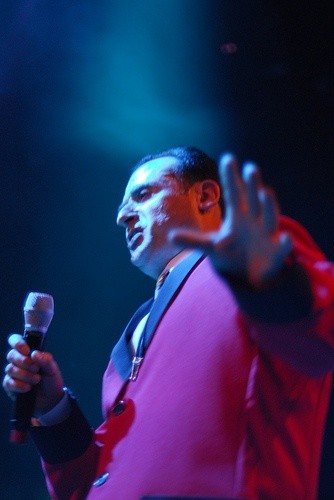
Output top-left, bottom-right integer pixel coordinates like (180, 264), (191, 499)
(9, 293), (54, 446)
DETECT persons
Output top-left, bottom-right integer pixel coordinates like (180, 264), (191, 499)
(3, 143), (334, 498)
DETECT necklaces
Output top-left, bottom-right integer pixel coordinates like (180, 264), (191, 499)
(127, 332), (147, 381)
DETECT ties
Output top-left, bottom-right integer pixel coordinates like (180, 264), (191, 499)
(158, 271), (169, 291)
